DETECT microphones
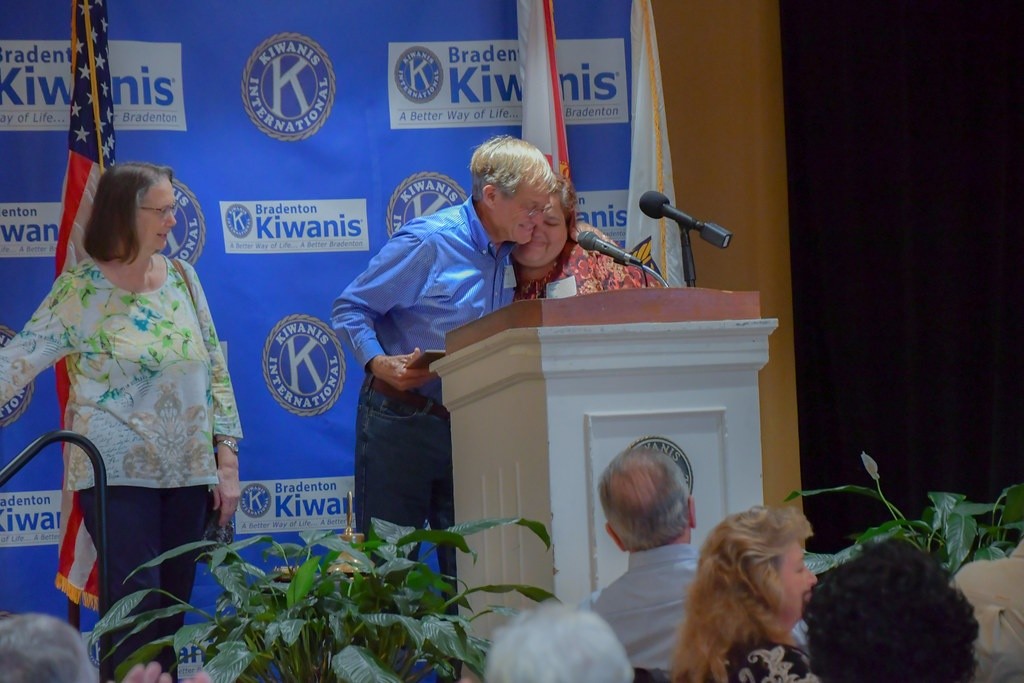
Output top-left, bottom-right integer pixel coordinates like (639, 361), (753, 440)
(577, 230), (642, 266)
(639, 190), (733, 248)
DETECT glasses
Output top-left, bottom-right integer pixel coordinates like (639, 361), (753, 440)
(137, 201), (179, 221)
(514, 196), (552, 219)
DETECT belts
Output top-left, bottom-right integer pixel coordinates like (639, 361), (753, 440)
(364, 374), (449, 420)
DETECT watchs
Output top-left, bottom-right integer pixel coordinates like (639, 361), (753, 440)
(218, 440), (239, 455)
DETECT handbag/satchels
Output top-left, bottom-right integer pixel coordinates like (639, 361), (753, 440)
(196, 453), (234, 552)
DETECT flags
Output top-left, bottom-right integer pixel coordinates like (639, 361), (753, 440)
(55, 0), (116, 611)
(517, 0), (571, 179)
(625, 0), (687, 287)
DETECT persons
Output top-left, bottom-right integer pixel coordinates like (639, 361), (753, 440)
(0, 613), (213, 683)
(592, 448), (701, 671)
(486, 601), (634, 683)
(510, 174), (662, 301)
(0, 161), (243, 683)
(332, 137), (558, 617)
(948, 538), (1024, 683)
(802, 538), (980, 683)
(673, 506), (817, 683)
